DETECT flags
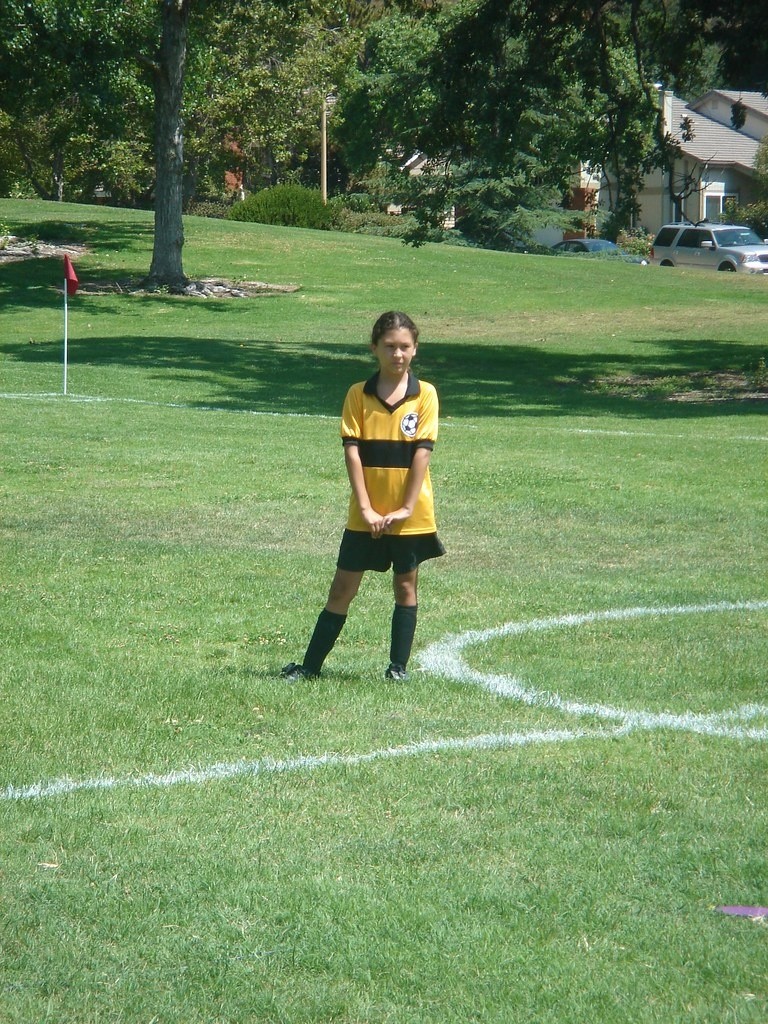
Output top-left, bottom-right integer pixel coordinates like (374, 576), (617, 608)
(64, 254), (78, 296)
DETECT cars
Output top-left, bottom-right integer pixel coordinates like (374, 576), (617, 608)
(549, 240), (648, 266)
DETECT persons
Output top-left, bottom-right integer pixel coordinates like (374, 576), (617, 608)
(280, 311), (447, 679)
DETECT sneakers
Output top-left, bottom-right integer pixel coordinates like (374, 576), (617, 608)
(385, 665), (412, 682)
(278, 663), (321, 684)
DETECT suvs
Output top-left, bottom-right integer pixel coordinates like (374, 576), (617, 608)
(650, 221), (768, 275)
